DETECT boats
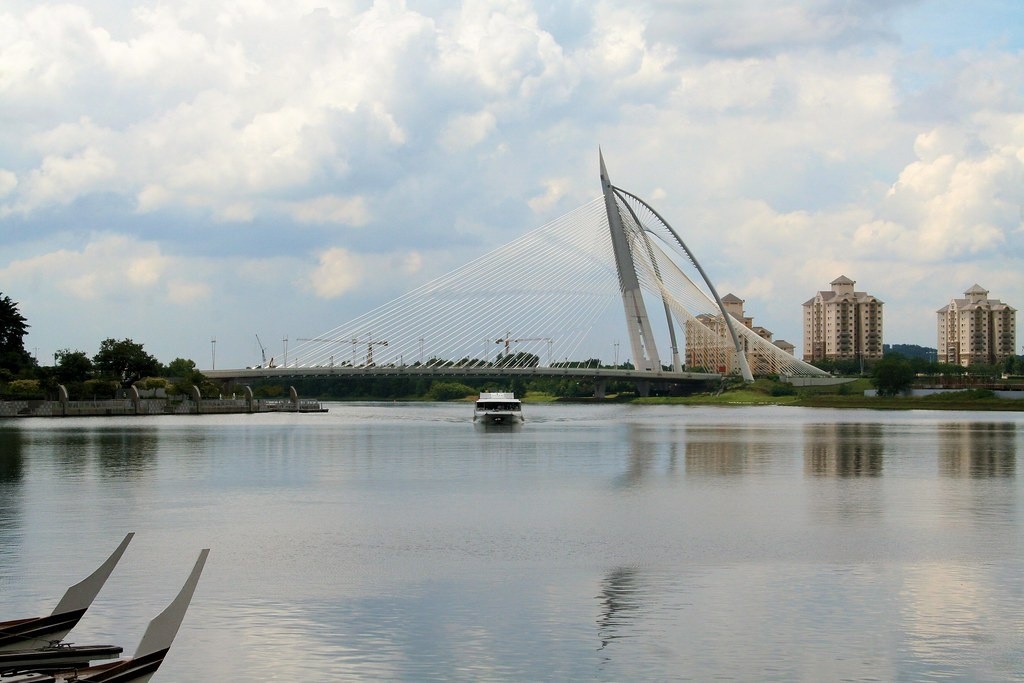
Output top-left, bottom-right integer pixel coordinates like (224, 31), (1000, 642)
(474, 388), (522, 424)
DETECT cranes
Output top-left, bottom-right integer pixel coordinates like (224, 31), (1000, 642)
(297, 332), (388, 368)
(496, 331), (552, 360)
